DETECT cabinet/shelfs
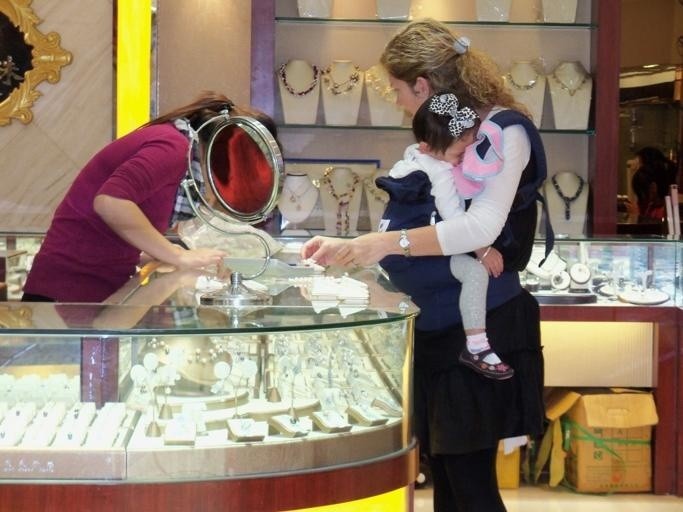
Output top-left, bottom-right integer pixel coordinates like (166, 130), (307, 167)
(253, 2), (622, 255)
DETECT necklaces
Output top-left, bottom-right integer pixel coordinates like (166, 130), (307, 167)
(285, 179), (305, 203)
(549, 174), (585, 219)
(506, 70), (540, 90)
(551, 70), (592, 97)
(278, 62), (320, 96)
(362, 175), (388, 204)
(322, 63), (361, 97)
(365, 65), (395, 101)
(324, 166), (360, 236)
(291, 182), (312, 211)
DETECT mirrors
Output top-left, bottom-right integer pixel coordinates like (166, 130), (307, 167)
(182, 108), (290, 313)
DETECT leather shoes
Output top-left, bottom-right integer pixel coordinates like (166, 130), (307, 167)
(459, 348), (514, 381)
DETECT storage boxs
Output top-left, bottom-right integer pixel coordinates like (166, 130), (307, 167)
(545, 389), (659, 496)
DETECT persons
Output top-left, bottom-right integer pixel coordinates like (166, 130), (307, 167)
(16, 89), (284, 303)
(629, 144), (682, 225)
(298, 13), (557, 512)
(371, 87), (515, 381)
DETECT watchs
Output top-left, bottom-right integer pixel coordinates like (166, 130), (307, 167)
(399, 228), (410, 257)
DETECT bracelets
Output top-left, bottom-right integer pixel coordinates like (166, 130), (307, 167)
(477, 246), (492, 264)
